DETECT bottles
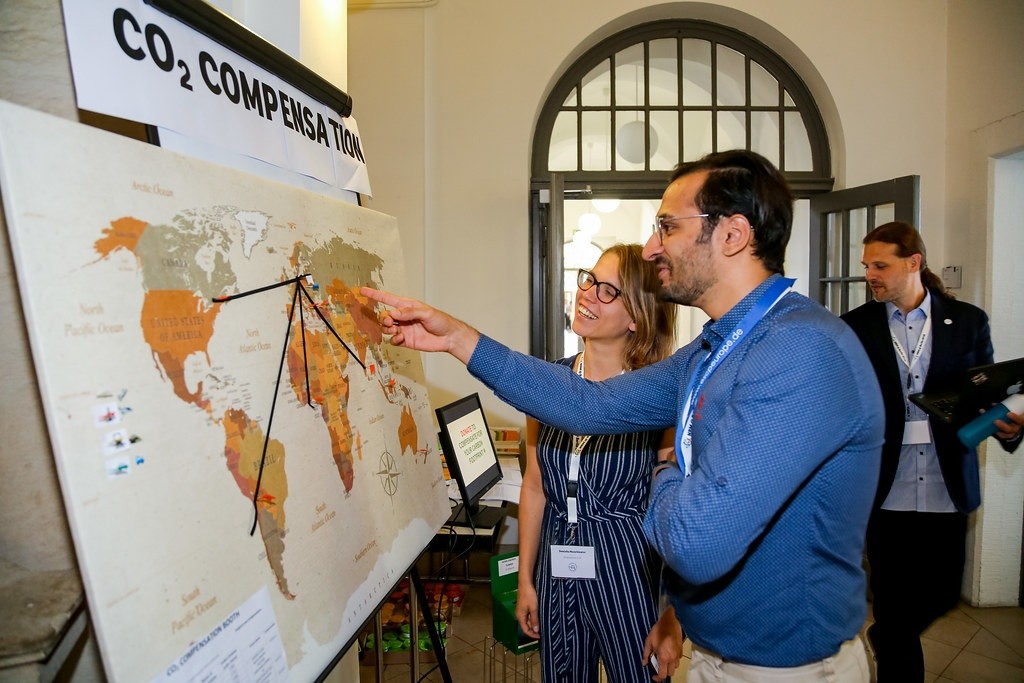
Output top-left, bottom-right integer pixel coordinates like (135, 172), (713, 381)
(366, 579), (462, 651)
(956, 391), (1024, 449)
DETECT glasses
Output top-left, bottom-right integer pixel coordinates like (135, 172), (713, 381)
(577, 267), (628, 304)
(656, 215), (755, 246)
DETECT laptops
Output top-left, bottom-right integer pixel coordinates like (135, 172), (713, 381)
(909, 357), (1024, 431)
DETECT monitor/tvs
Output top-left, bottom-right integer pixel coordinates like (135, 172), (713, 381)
(435, 391), (509, 530)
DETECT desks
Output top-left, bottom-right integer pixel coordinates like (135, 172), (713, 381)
(428, 446), (521, 583)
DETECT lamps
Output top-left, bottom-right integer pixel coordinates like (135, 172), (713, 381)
(615, 63), (659, 162)
(562, 197), (620, 264)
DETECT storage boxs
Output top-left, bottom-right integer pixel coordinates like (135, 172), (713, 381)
(356, 584), (469, 665)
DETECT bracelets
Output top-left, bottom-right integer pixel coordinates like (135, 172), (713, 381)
(652, 460), (680, 480)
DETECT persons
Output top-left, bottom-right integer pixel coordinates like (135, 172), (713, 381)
(838, 222), (1024, 683)
(360, 149), (886, 683)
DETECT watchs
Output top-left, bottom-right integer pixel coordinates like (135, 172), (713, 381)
(1004, 433), (1021, 442)
